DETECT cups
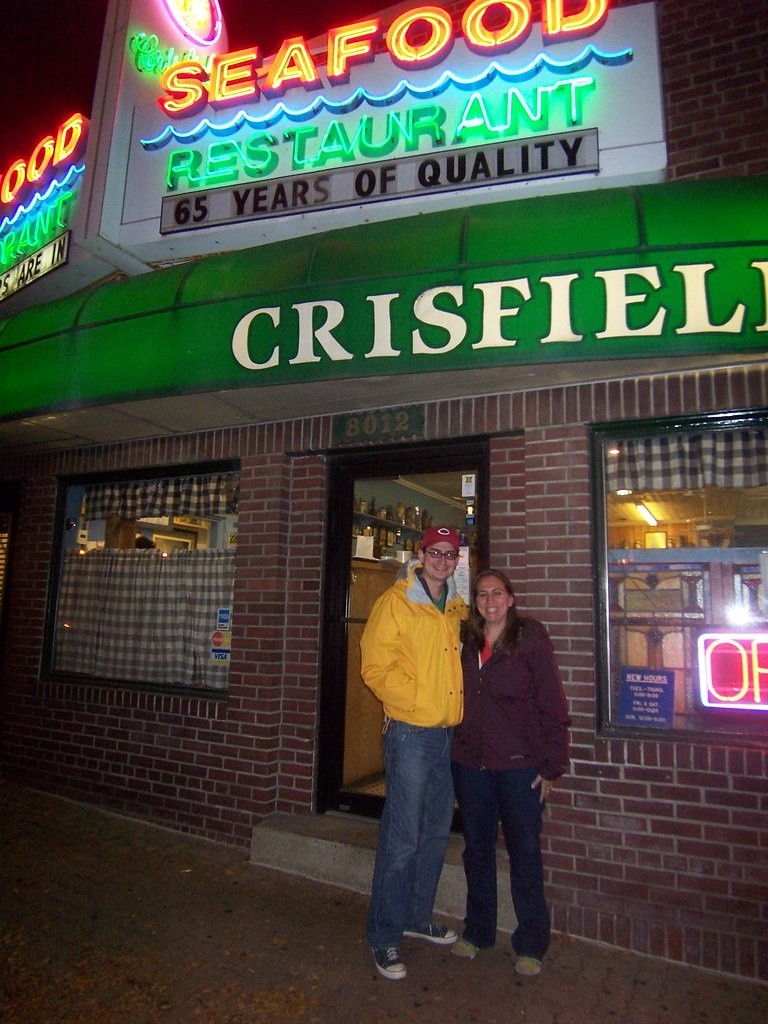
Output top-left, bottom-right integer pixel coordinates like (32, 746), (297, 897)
(396, 551), (412, 563)
(380, 555), (393, 560)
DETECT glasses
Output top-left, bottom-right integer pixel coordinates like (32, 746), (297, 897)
(424, 549), (459, 560)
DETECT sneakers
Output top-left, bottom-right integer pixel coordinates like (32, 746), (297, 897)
(403, 920), (460, 946)
(370, 942), (407, 981)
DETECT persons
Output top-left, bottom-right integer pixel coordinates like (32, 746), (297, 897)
(360, 526), (474, 979)
(451, 568), (571, 975)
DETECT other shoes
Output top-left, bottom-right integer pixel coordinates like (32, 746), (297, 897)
(515, 955), (542, 976)
(450, 937), (481, 960)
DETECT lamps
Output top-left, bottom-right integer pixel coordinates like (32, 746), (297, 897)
(635, 500), (658, 527)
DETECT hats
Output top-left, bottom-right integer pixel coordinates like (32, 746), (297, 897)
(421, 524), (460, 551)
